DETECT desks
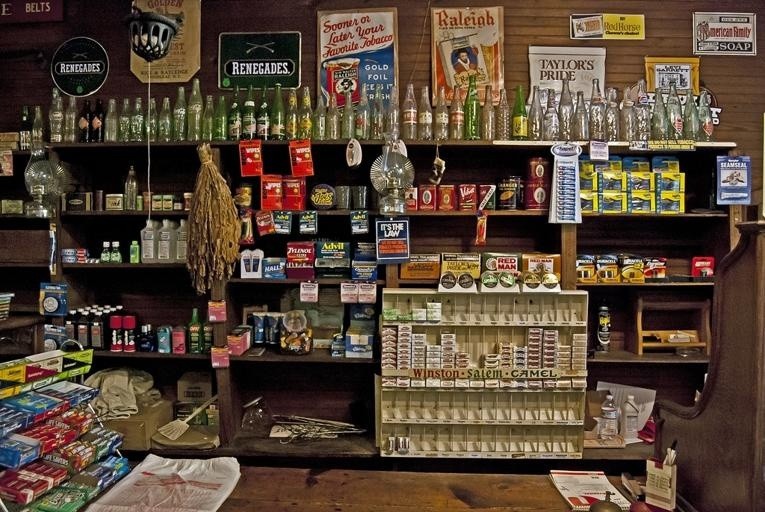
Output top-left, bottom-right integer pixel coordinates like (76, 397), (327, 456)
(80, 466), (647, 512)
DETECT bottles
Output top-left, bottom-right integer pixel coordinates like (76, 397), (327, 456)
(598, 390), (641, 441)
(595, 301), (614, 355)
(64, 302), (214, 356)
(122, 163), (141, 211)
(99, 217), (201, 263)
(17, 71), (714, 152)
(241, 400), (273, 439)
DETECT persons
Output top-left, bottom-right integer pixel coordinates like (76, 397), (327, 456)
(453, 48), (477, 74)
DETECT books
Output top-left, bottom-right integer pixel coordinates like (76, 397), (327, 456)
(548, 468), (632, 512)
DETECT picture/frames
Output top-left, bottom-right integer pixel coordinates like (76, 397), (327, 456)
(218, 30), (302, 91)
(431, 6), (505, 106)
(317, 6), (400, 121)
(692, 12), (756, 57)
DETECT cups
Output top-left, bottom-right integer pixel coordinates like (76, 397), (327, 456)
(334, 184), (379, 211)
